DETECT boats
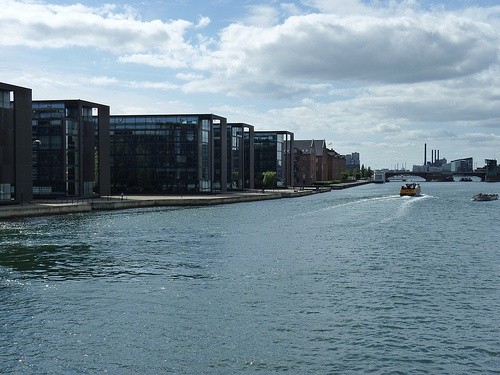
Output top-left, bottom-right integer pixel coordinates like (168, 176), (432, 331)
(459, 177), (472, 181)
(400, 183), (421, 196)
(473, 192), (499, 201)
